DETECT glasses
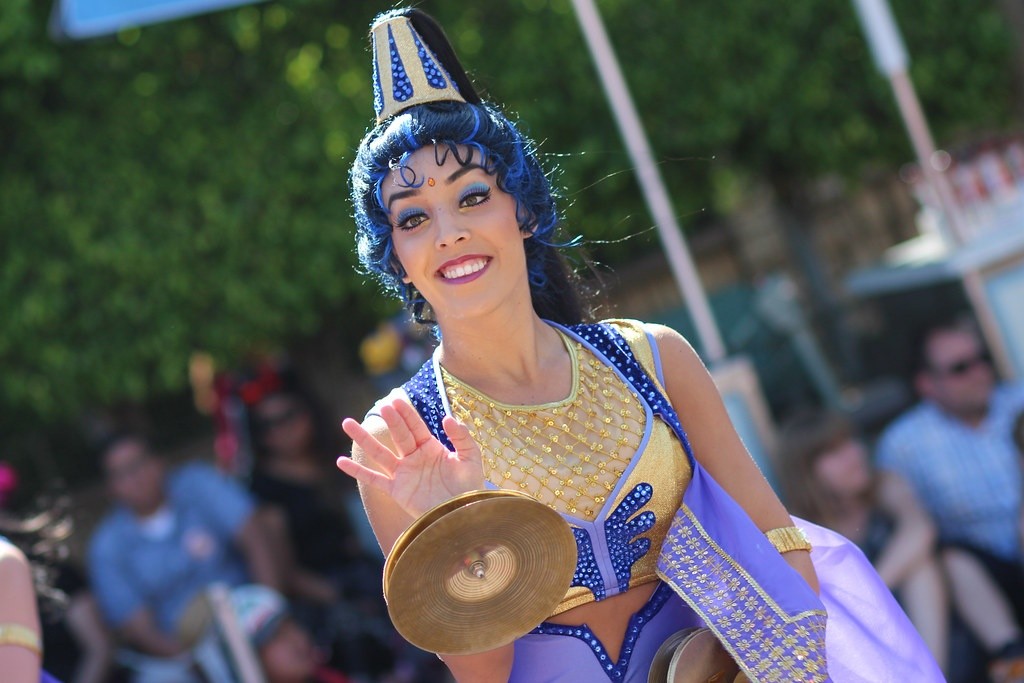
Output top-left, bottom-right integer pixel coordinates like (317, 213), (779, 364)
(928, 353), (987, 375)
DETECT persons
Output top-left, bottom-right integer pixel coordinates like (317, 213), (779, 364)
(1, 368), (441, 682)
(775, 316), (1024, 682)
(335, 6), (822, 683)
